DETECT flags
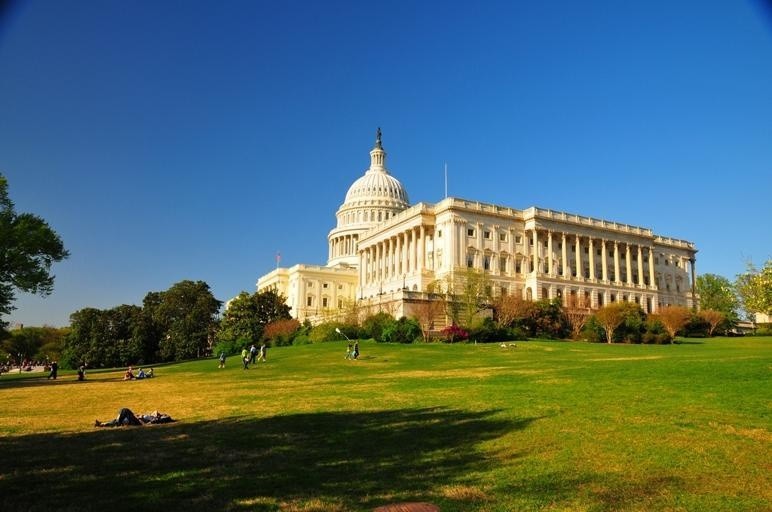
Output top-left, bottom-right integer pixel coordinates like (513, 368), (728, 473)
(276, 252), (281, 263)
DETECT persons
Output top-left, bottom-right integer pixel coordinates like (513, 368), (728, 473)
(241, 345), (248, 367)
(243, 356), (250, 369)
(217, 352), (226, 368)
(352, 340), (359, 359)
(258, 343), (267, 362)
(0, 357), (86, 382)
(95, 408), (172, 427)
(344, 340), (354, 360)
(250, 344), (257, 364)
(122, 365), (154, 382)
